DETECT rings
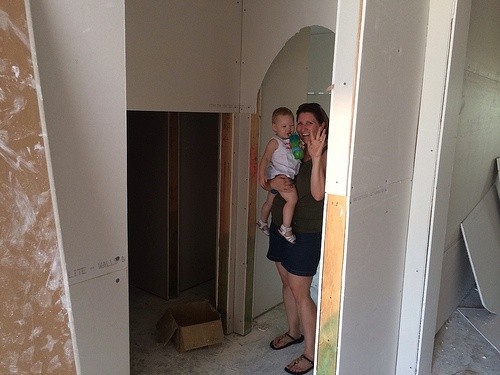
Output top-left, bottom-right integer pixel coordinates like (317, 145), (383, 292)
(319, 139), (322, 141)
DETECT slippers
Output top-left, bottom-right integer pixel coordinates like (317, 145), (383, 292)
(269, 332), (305, 351)
(284, 353), (314, 375)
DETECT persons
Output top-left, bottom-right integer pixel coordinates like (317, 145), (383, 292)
(256, 107), (305, 244)
(262, 103), (329, 375)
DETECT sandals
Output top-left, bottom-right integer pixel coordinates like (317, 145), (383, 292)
(256, 218), (271, 236)
(277, 224), (297, 244)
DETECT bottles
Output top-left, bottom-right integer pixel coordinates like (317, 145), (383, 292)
(289, 133), (304, 160)
